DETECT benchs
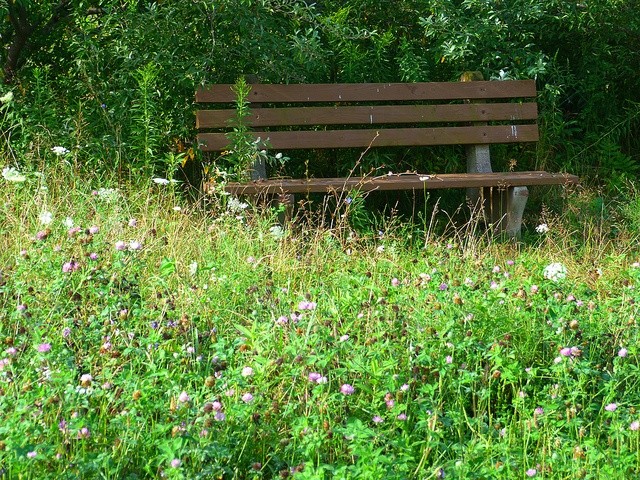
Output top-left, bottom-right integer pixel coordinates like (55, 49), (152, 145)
(196, 72), (578, 242)
